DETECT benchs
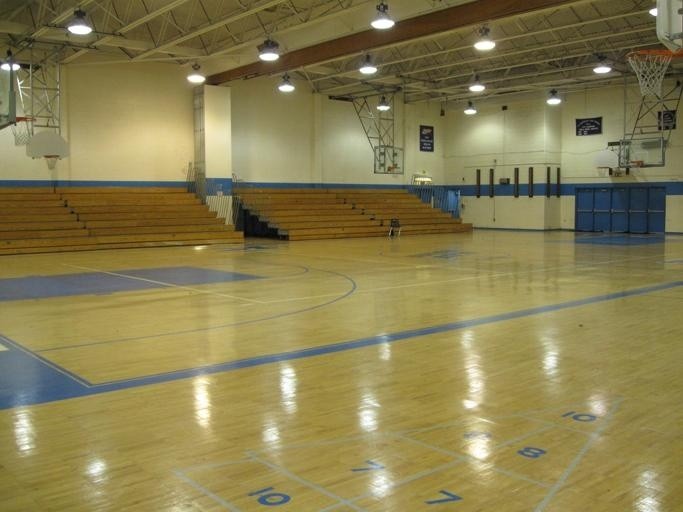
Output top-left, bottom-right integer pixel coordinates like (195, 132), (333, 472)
(2, 186), (245, 253)
(232, 186), (472, 241)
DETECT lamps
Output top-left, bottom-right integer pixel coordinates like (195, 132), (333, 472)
(0, 44), (19, 72)
(412, 175), (432, 185)
(439, 22), (613, 116)
(183, 1), (400, 112)
(67, 3), (91, 36)
